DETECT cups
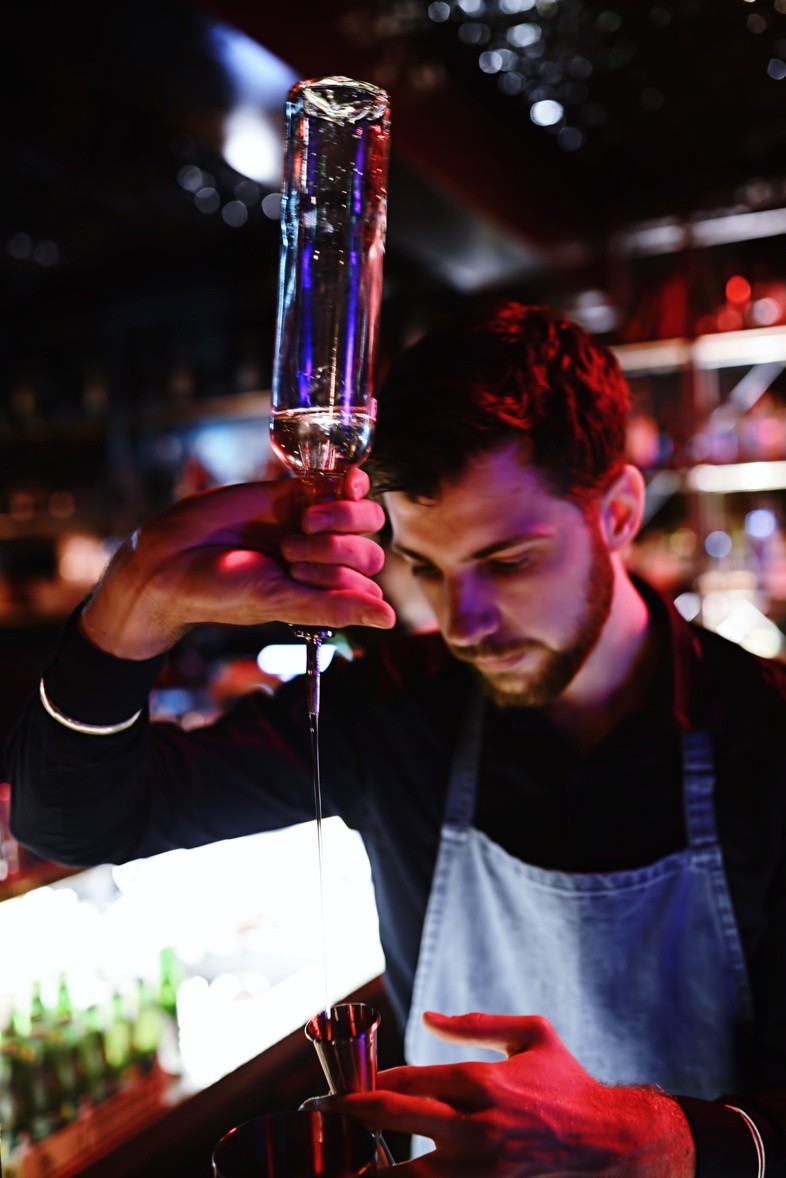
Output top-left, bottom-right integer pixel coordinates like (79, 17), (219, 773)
(306, 1004), (380, 1097)
(212, 1109), (397, 1178)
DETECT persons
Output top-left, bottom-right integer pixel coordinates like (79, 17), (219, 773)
(10, 294), (786, 1178)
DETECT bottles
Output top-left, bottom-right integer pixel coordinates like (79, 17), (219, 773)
(268, 74), (391, 730)
(0, 956), (181, 1158)
